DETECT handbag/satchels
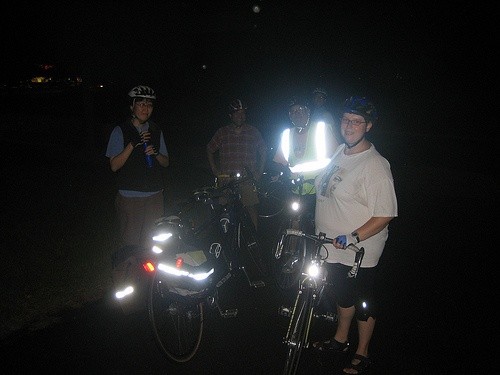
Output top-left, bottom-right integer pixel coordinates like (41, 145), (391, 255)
(165, 228), (234, 311)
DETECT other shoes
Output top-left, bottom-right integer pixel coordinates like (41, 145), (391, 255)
(119, 299), (137, 315)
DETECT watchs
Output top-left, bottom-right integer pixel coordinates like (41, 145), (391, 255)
(351, 231), (359, 244)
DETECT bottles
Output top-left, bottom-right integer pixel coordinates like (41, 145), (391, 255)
(144, 141), (153, 169)
(219, 208), (232, 233)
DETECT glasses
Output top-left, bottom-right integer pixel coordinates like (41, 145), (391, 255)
(339, 117), (369, 126)
(136, 103), (153, 109)
(291, 106), (308, 113)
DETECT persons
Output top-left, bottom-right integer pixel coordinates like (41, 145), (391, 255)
(105, 84), (174, 323)
(206, 100), (267, 232)
(312, 95), (398, 375)
(271, 89), (340, 219)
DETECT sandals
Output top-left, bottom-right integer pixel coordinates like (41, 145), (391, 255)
(312, 336), (352, 354)
(339, 351), (372, 375)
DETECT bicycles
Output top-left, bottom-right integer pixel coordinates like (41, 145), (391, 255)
(140, 169), (318, 365)
(269, 228), (366, 375)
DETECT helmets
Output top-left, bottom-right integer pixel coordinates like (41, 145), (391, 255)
(338, 95), (378, 126)
(311, 87), (329, 97)
(230, 99), (248, 113)
(288, 94), (311, 109)
(128, 86), (156, 100)
